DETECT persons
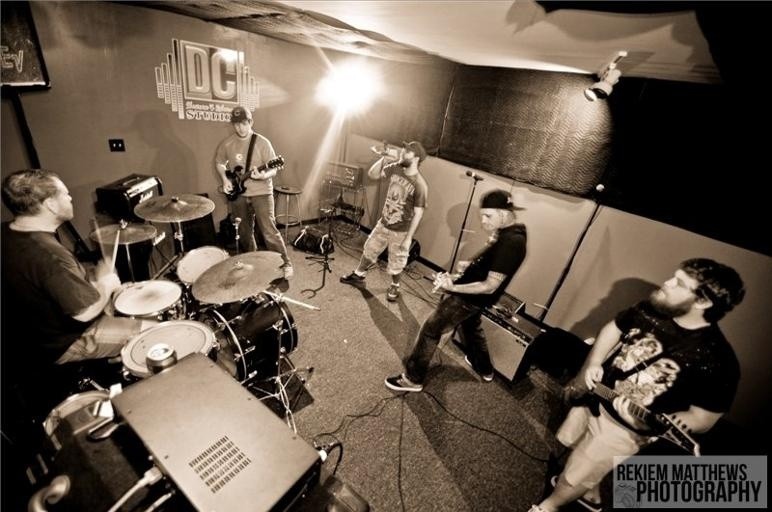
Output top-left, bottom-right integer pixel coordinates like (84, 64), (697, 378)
(0, 169), (163, 367)
(526, 259), (744, 512)
(340, 142), (429, 302)
(385, 191), (527, 391)
(217, 107), (293, 282)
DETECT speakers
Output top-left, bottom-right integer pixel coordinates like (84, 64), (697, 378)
(452, 303), (546, 384)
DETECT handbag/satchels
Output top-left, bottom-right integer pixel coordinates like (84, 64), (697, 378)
(293, 226), (334, 256)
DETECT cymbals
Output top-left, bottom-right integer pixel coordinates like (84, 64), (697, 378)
(190, 250), (286, 305)
(133, 191), (216, 224)
(88, 219), (159, 245)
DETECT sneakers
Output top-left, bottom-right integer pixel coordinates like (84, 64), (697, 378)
(385, 373), (423, 391)
(285, 267), (293, 280)
(340, 270), (365, 283)
(551, 475), (602, 512)
(387, 285), (400, 301)
(465, 355), (494, 381)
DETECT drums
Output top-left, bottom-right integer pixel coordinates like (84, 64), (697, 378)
(117, 318), (220, 382)
(185, 291), (299, 386)
(113, 279), (190, 322)
(176, 245), (233, 290)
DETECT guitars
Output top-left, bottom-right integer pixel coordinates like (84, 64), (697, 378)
(220, 155), (286, 202)
(562, 359), (705, 456)
(433, 258), (470, 292)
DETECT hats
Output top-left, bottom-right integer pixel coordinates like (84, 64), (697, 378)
(231, 106), (252, 122)
(402, 141), (425, 162)
(480, 189), (527, 211)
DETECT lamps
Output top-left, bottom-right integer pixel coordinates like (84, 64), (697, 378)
(586, 52), (628, 102)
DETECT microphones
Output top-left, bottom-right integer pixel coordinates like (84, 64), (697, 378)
(380, 151), (403, 162)
(463, 169), (484, 184)
(596, 184), (614, 205)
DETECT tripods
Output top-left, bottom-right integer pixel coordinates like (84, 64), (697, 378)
(423, 176), (478, 305)
(304, 214), (344, 273)
